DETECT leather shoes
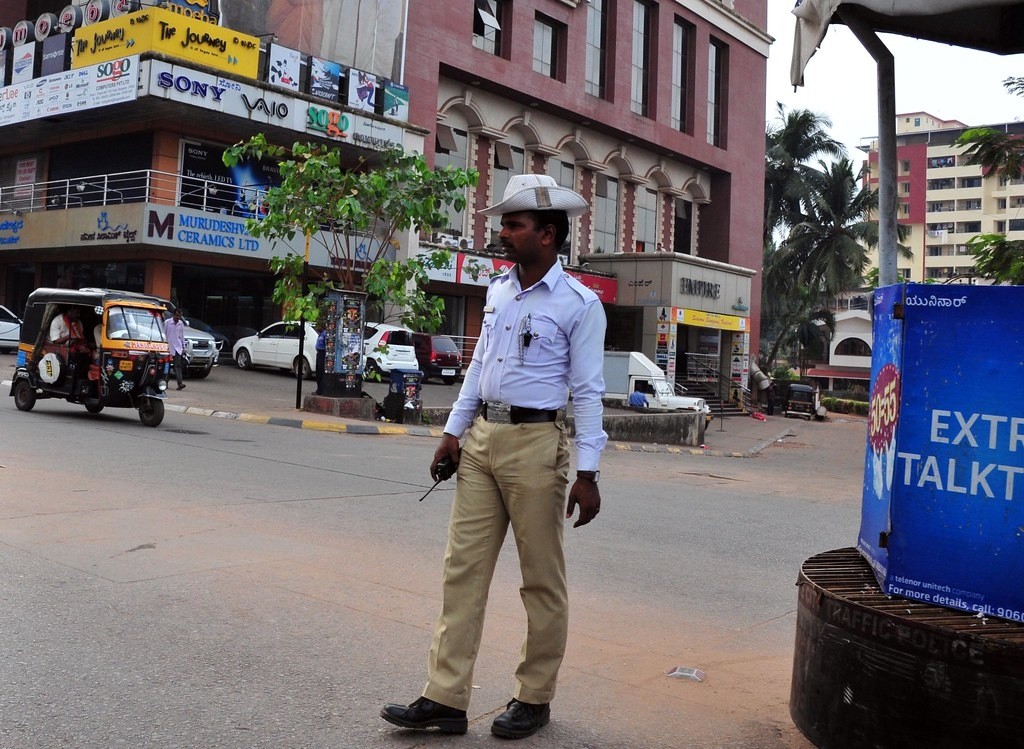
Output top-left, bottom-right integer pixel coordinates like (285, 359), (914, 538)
(491, 697), (550, 739)
(380, 696), (467, 734)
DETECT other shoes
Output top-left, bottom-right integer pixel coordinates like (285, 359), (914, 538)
(176, 384), (186, 389)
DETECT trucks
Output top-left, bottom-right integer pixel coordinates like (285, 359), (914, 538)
(569, 351), (713, 431)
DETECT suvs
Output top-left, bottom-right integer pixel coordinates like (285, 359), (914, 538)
(111, 314), (224, 379)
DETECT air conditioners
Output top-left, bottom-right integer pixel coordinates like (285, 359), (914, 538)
(436, 231), (474, 251)
(557, 254), (568, 266)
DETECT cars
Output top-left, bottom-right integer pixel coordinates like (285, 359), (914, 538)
(362, 322), (419, 382)
(212, 326), (261, 349)
(232, 321), (367, 382)
(0, 304), (24, 353)
(184, 316), (230, 350)
(411, 332), (463, 385)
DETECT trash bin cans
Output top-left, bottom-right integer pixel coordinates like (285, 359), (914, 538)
(388, 368), (423, 409)
(785, 384), (814, 419)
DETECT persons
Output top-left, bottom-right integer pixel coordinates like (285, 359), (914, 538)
(628, 383), (650, 408)
(311, 319), (326, 395)
(379, 173), (609, 740)
(50, 306), (90, 404)
(163, 307), (186, 391)
(93, 312), (128, 366)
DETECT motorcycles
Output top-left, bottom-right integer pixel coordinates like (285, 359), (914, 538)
(785, 384), (813, 421)
(10, 288), (174, 427)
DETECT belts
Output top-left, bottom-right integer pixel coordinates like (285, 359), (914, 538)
(480, 400), (558, 425)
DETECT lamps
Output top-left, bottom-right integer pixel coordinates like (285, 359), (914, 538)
(659, 308), (667, 321)
(76, 181), (123, 203)
(178, 184), (219, 203)
(738, 297), (743, 303)
(127, 1), (156, 11)
(54, 21), (81, 32)
(254, 32), (279, 44)
(51, 194), (84, 207)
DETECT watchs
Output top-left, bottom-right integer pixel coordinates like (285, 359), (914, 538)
(576, 470), (600, 483)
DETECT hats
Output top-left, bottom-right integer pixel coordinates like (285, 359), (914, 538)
(476, 174), (590, 217)
